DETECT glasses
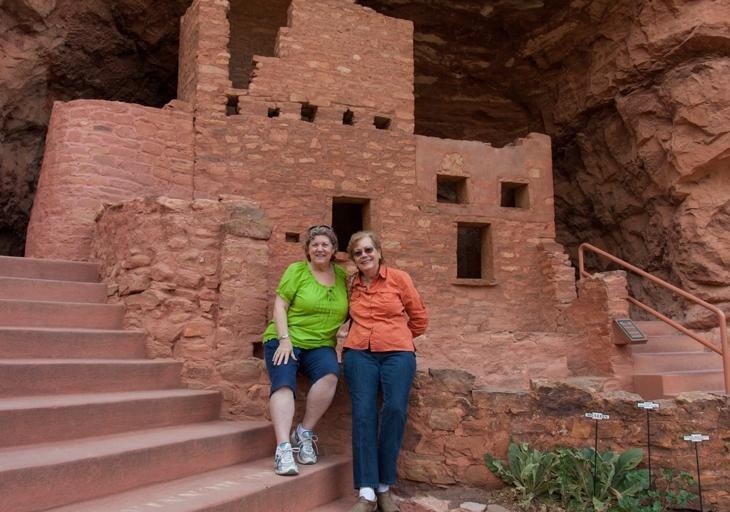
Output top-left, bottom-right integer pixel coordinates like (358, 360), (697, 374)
(351, 246), (374, 258)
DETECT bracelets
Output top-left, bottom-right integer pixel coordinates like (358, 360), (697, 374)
(278, 334), (288, 340)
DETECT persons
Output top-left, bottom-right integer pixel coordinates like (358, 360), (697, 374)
(262, 224), (352, 477)
(341, 229), (429, 511)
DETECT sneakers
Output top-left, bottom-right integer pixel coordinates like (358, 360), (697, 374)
(274, 423), (320, 475)
(347, 490), (402, 512)
